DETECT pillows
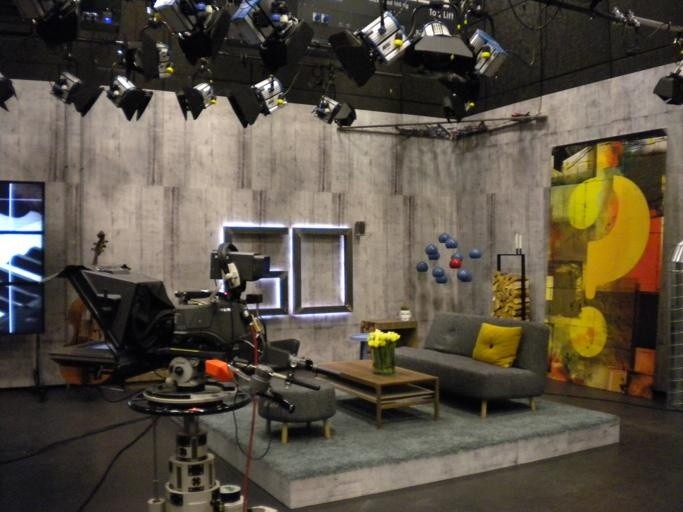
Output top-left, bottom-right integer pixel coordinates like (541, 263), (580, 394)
(471, 321), (523, 370)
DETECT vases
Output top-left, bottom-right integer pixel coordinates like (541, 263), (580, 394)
(370, 342), (397, 376)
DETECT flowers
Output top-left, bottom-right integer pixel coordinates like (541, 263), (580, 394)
(367, 327), (401, 349)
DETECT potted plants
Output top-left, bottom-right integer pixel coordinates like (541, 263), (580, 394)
(398, 305), (412, 321)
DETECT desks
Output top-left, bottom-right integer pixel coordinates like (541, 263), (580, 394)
(350, 332), (368, 358)
(359, 317), (418, 353)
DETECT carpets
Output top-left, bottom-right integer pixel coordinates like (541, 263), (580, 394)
(175, 388), (620, 482)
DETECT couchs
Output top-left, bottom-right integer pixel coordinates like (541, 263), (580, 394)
(258, 331), (336, 443)
(389, 312), (551, 418)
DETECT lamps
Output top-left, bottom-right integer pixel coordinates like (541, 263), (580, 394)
(653, 22), (682, 108)
(1, 1), (510, 128)
